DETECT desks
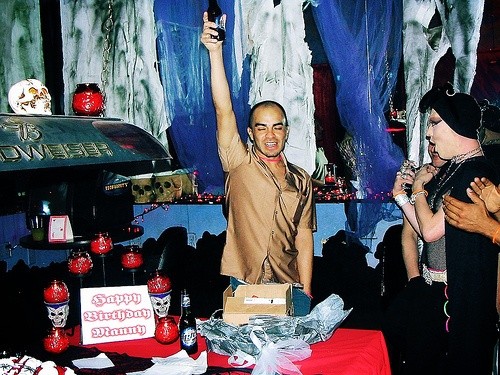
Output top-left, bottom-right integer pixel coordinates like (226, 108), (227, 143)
(64, 315), (390, 375)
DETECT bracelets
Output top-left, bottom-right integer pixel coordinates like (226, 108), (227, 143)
(493, 226), (500, 244)
(409, 190), (429, 205)
(393, 189), (412, 207)
(305, 291), (314, 299)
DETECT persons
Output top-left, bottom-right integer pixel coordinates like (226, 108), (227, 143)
(401, 136), (447, 283)
(392, 83), (500, 375)
(442, 177), (500, 375)
(200, 10), (317, 318)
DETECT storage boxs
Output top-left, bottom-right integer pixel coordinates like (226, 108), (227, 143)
(221, 282), (292, 323)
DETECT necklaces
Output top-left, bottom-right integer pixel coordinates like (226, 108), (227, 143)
(260, 157), (282, 162)
(428, 145), (482, 210)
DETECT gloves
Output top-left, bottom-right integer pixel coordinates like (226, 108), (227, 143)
(410, 276), (428, 299)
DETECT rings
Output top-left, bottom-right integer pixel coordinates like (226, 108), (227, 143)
(402, 175), (407, 179)
(400, 168), (406, 175)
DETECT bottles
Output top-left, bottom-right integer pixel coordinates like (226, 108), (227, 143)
(180, 296), (197, 354)
(43, 278), (70, 303)
(206, 0), (226, 41)
(68, 251), (92, 273)
(147, 269), (170, 294)
(154, 317), (180, 344)
(72, 83), (103, 116)
(43, 328), (69, 354)
(121, 246), (142, 269)
(90, 232), (113, 254)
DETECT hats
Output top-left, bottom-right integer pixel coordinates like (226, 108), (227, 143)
(480, 98), (500, 133)
(418, 81), (482, 140)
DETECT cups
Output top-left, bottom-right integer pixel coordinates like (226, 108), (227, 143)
(31, 228), (45, 241)
(119, 269), (145, 284)
(323, 163), (336, 186)
(94, 255), (116, 286)
(43, 301), (72, 328)
(71, 275), (92, 325)
(149, 290), (175, 317)
